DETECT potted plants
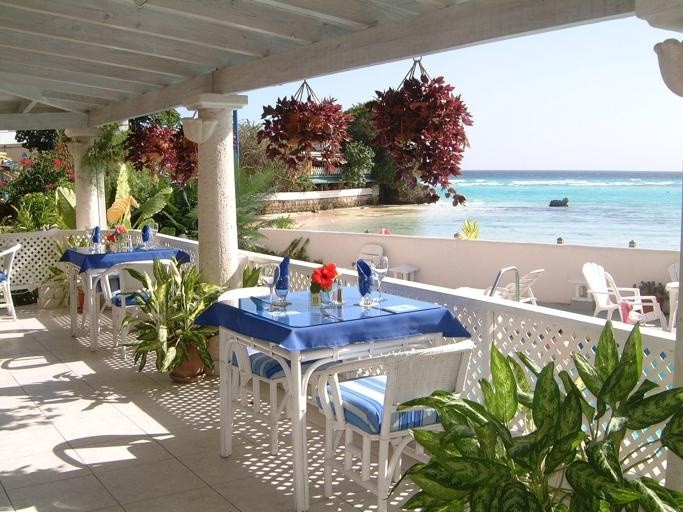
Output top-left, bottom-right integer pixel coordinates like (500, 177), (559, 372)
(632, 281), (669, 314)
(121, 122), (176, 176)
(168, 125), (198, 188)
(41, 236), (91, 313)
(256, 95), (353, 176)
(364, 75), (473, 207)
(115, 255), (230, 383)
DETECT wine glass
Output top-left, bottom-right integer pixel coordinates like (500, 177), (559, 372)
(275, 276), (290, 306)
(87, 233), (152, 253)
(261, 263), (281, 310)
(357, 276), (372, 307)
(370, 255), (388, 303)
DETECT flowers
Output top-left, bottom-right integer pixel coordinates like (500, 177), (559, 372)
(106, 225), (127, 242)
(310, 263), (337, 293)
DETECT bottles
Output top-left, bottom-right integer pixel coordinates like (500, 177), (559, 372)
(309, 281), (321, 307)
(331, 279), (342, 303)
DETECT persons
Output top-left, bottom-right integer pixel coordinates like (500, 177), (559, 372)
(0, 197), (7, 221)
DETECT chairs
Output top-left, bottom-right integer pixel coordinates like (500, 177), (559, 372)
(93, 259), (171, 361)
(315, 339), (474, 512)
(582, 263), (644, 327)
(217, 287), (343, 456)
(0, 243), (21, 322)
(352, 245), (388, 277)
(604, 271), (667, 333)
(483, 269), (544, 305)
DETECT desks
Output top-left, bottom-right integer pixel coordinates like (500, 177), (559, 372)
(665, 282), (679, 333)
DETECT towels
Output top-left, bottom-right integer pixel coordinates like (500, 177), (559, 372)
(356, 259), (373, 295)
(92, 226), (101, 243)
(274, 256), (290, 290)
(141, 225), (150, 242)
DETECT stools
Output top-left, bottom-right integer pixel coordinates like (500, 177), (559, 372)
(388, 264), (418, 282)
